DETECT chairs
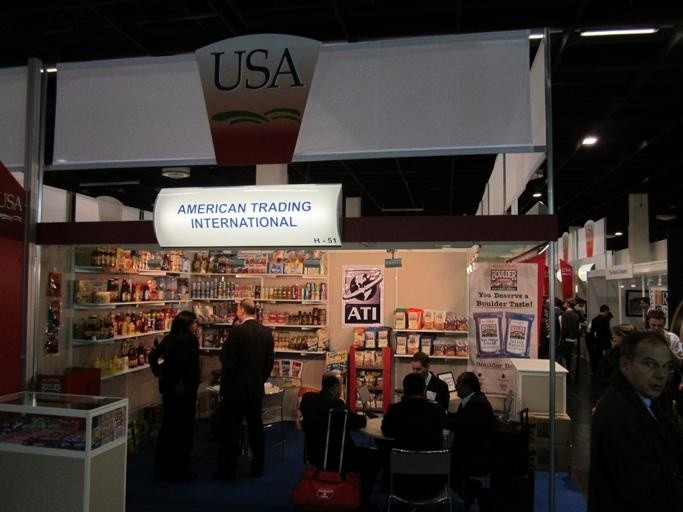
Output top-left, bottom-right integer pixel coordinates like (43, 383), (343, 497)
(300, 390), (533, 511)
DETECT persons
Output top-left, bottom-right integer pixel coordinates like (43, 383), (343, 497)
(299, 372), (366, 472)
(538, 294), (682, 512)
(443, 371), (507, 501)
(411, 352), (449, 413)
(210, 298), (275, 480)
(147, 311), (201, 483)
(380, 372), (449, 512)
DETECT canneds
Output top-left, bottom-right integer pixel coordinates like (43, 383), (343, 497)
(192, 278), (234, 298)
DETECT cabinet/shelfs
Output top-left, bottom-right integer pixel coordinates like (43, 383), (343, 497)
(347, 326), (467, 414)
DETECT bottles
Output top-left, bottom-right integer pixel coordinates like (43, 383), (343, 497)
(111, 310), (165, 335)
(137, 343), (146, 364)
(120, 278), (129, 301)
(191, 279), (327, 302)
(297, 311), (313, 325)
(93, 355), (121, 377)
(93, 247), (117, 267)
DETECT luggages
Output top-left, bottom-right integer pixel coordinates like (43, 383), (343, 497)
(508, 408), (534, 512)
(292, 408), (362, 511)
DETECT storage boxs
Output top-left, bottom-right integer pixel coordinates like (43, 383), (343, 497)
(524, 412), (572, 471)
